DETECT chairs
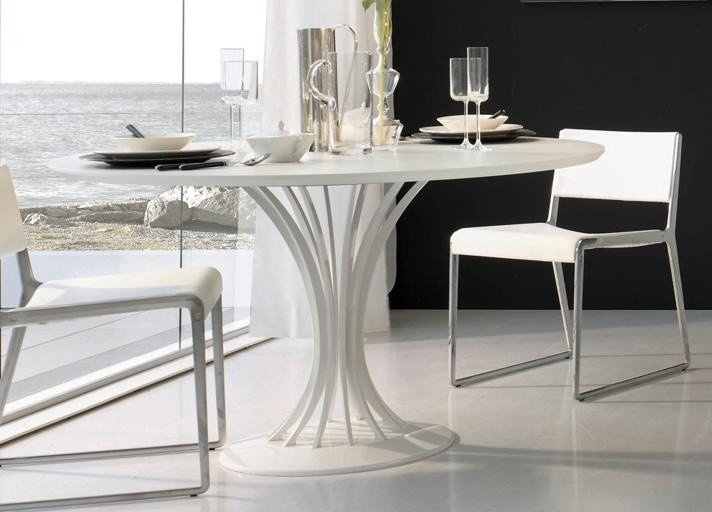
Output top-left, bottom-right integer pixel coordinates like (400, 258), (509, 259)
(448, 129), (690, 400)
(1, 165), (226, 511)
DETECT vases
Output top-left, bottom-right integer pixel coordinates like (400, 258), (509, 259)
(366, 1), (404, 150)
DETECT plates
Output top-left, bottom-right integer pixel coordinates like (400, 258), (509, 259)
(78, 148), (236, 168)
(412, 124), (524, 144)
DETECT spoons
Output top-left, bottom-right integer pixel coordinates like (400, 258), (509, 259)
(155, 152), (272, 170)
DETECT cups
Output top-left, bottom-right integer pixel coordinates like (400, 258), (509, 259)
(305, 51), (374, 155)
(296, 24), (361, 154)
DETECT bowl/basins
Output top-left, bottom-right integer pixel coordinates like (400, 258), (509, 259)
(435, 115), (508, 133)
(110, 134), (198, 150)
(247, 132), (316, 163)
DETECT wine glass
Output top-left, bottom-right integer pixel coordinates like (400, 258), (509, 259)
(219, 44), (258, 157)
(446, 44), (493, 154)
